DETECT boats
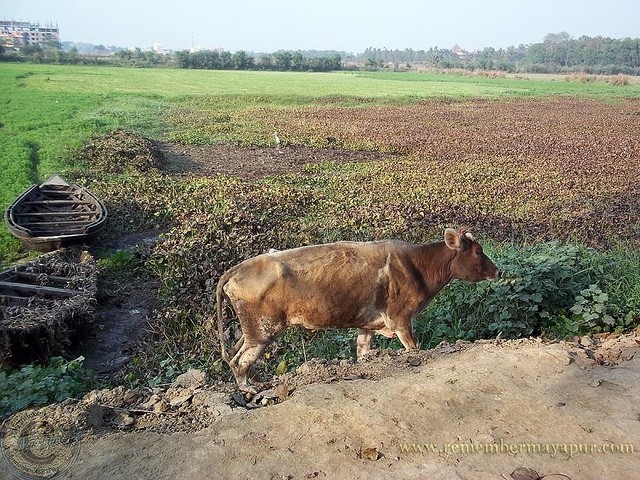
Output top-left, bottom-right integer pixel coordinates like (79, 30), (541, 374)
(5, 174), (108, 251)
(0, 243), (99, 362)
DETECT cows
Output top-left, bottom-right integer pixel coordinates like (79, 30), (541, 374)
(215, 227), (501, 393)
